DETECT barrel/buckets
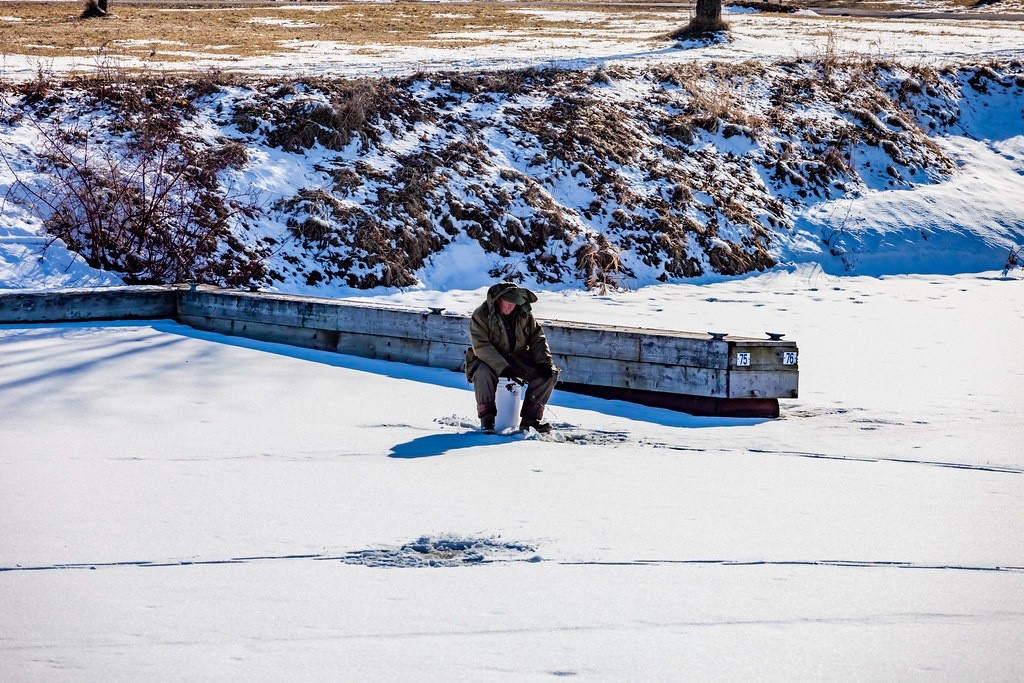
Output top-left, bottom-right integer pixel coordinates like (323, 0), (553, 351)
(493, 377), (524, 430)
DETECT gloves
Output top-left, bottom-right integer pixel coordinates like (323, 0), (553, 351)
(537, 363), (553, 380)
(500, 366), (526, 379)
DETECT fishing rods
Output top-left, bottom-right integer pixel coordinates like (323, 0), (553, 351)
(514, 378), (580, 437)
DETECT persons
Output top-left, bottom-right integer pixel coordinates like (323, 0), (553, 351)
(465, 282), (561, 434)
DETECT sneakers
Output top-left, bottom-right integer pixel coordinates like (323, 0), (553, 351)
(519, 416), (553, 433)
(481, 416), (495, 433)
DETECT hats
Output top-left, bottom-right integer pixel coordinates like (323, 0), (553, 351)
(502, 288), (526, 305)
